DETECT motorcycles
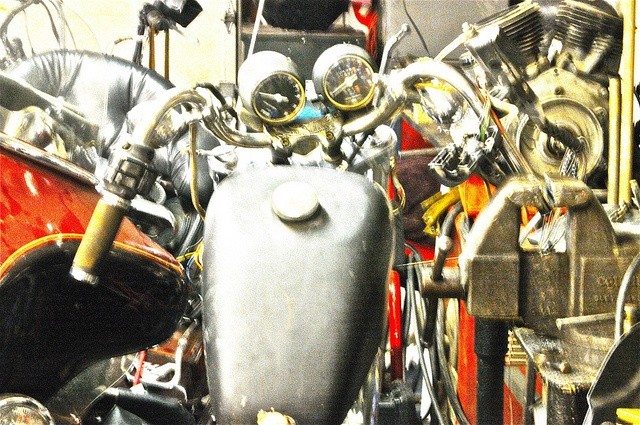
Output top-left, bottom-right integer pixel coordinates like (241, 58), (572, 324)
(66, 21), (502, 425)
